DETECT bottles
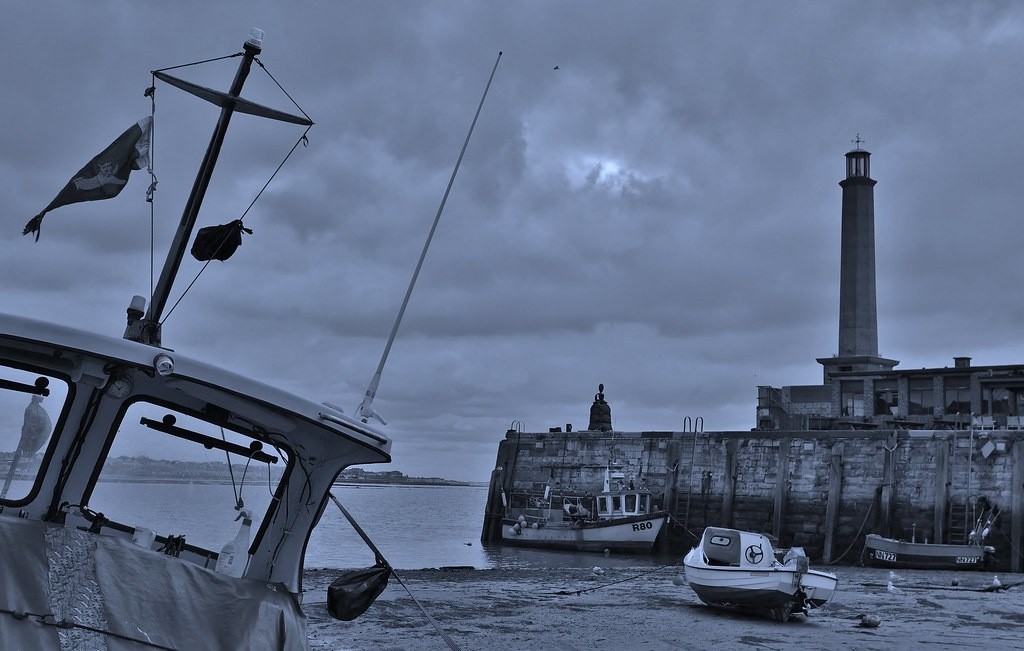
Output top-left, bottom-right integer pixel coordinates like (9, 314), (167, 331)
(215, 519), (252, 578)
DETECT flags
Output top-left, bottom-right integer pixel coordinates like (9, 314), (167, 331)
(22, 116), (151, 245)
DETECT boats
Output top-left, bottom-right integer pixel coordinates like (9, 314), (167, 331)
(684, 524), (839, 622)
(864, 502), (1004, 572)
(501, 460), (670, 557)
(1, 25), (503, 651)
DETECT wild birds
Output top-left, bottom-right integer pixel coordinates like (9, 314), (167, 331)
(888, 570), (908, 583)
(992, 575), (1001, 587)
(887, 582), (904, 596)
(554, 65), (559, 71)
(592, 565), (605, 576)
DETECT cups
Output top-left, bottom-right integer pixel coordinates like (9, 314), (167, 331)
(132, 525), (157, 550)
